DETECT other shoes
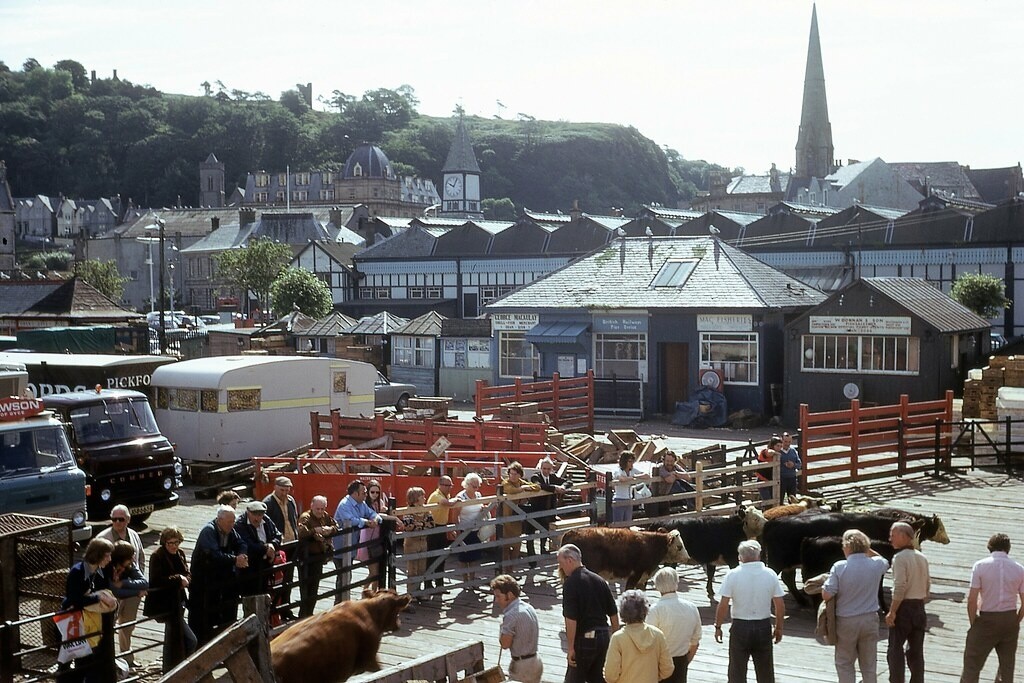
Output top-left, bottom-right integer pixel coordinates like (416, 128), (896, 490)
(417, 595), (428, 601)
(128, 661), (144, 669)
(443, 590), (451, 594)
(529, 561), (536, 569)
(541, 547), (551, 555)
(281, 615), (298, 622)
(410, 597), (418, 603)
(512, 570), (522, 581)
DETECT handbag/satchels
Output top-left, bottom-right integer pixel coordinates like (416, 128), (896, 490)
(52, 610), (93, 664)
(633, 481), (652, 499)
(477, 511), (496, 541)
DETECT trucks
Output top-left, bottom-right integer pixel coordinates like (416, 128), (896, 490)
(0, 398), (93, 567)
(1, 362), (185, 529)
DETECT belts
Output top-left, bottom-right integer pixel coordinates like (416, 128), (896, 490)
(512, 652), (536, 661)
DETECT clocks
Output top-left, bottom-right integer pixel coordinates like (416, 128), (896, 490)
(445, 176), (462, 197)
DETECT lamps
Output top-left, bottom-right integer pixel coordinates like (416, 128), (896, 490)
(585, 327), (590, 338)
(381, 335), (387, 346)
(238, 337), (244, 346)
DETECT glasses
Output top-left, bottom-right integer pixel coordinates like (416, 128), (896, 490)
(111, 517), (127, 523)
(469, 484), (482, 489)
(367, 490), (380, 494)
(165, 540), (180, 547)
(440, 483), (453, 488)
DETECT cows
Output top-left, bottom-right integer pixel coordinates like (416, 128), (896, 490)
(640, 492), (952, 618)
(557, 526), (693, 594)
(270, 586), (418, 683)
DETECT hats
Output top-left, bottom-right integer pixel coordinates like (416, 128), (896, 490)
(274, 476), (293, 487)
(246, 500), (268, 513)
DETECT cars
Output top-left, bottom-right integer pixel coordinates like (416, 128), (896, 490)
(145, 310), (248, 342)
(373, 369), (425, 410)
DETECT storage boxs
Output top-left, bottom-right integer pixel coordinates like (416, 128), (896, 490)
(944, 355), (1024, 465)
(297, 397), (725, 553)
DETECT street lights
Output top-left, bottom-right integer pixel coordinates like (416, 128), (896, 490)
(144, 214), (170, 357)
(135, 237), (166, 313)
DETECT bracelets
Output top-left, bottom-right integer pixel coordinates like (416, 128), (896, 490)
(714, 623), (721, 629)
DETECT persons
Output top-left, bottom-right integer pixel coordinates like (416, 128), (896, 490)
(754, 431), (802, 511)
(402, 472), (491, 602)
(55, 537), (118, 672)
(355, 479), (403, 593)
(960, 532), (1024, 683)
(102, 540), (149, 598)
(492, 539), (785, 683)
(148, 525), (197, 676)
(188, 503), (249, 652)
(499, 455), (573, 577)
(217, 490), (241, 510)
(96, 504), (146, 667)
(822, 522), (931, 683)
(331, 479), (382, 606)
(297, 495), (339, 620)
(613, 450), (691, 528)
(262, 476), (298, 621)
(234, 500), (282, 620)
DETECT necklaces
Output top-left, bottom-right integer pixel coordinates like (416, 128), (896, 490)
(89, 573), (97, 588)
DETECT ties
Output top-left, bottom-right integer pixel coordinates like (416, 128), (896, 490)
(546, 478), (548, 484)
